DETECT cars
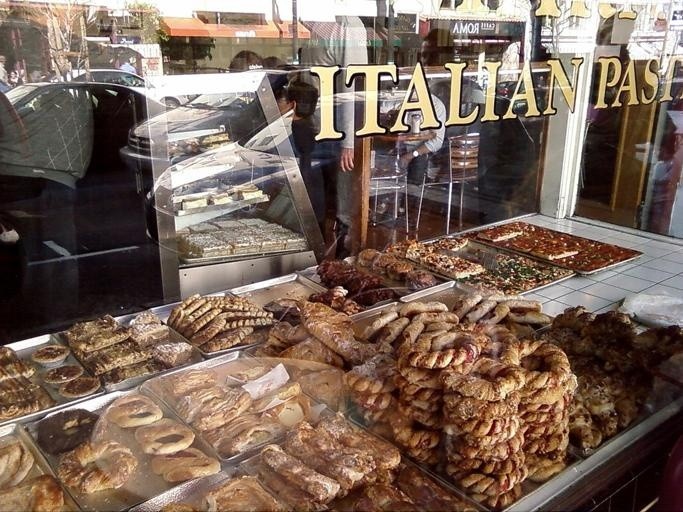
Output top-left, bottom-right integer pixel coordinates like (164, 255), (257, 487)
(5, 67), (180, 110)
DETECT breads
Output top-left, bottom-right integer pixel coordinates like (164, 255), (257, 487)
(476, 249), (575, 295)
(358, 249), (436, 292)
(38, 408), (100, 456)
(159, 500), (202, 512)
(277, 295), (683, 511)
(43, 365), (84, 384)
(180, 223), (307, 258)
(152, 447), (220, 482)
(466, 222), (637, 272)
(135, 417), (195, 456)
(129, 312), (193, 371)
(58, 377), (101, 397)
(32, 344), (70, 363)
(258, 410), (401, 512)
(0, 474), (64, 512)
(206, 475), (289, 512)
(64, 314), (153, 388)
(58, 438), (137, 493)
(105, 394), (162, 428)
(166, 293), (273, 352)
(0, 347), (57, 420)
(386, 238), (483, 279)
(174, 184), (263, 210)
(0, 440), (34, 490)
(150, 367), (305, 455)
(353, 466), (479, 512)
(176, 218), (268, 244)
(262, 286), (360, 326)
(170, 133), (240, 165)
(317, 258), (394, 306)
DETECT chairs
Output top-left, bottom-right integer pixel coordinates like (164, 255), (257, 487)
(369, 128), (482, 242)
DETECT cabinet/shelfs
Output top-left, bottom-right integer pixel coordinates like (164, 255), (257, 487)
(109, 55), (330, 298)
